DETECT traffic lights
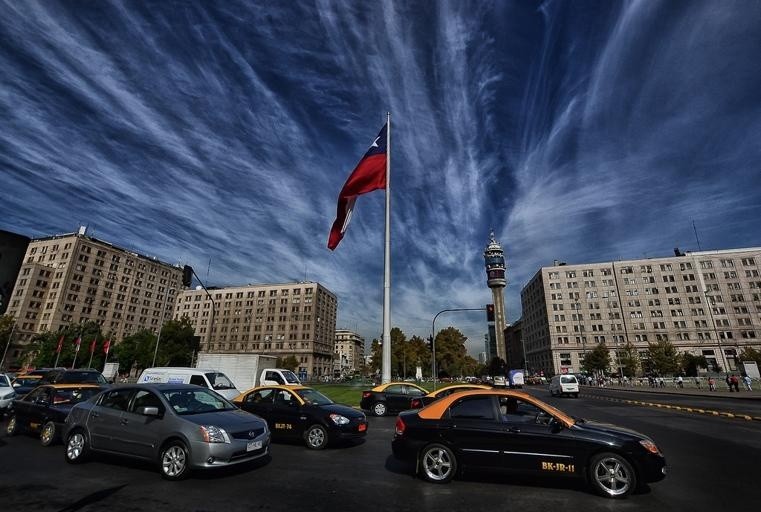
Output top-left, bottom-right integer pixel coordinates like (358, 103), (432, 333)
(487, 303), (495, 322)
(427, 333), (434, 354)
(179, 264), (193, 287)
(526, 361), (530, 371)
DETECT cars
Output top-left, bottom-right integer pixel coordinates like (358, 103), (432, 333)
(308, 369), (547, 389)
(0, 367), (112, 418)
(61, 383), (272, 481)
(391, 389), (668, 498)
(360, 381), (432, 417)
(410, 384), (499, 409)
(3, 384), (103, 447)
(228, 385), (369, 450)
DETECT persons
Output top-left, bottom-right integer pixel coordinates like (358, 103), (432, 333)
(501, 402), (515, 422)
(320, 372), (511, 387)
(648, 375), (665, 388)
(727, 373), (752, 393)
(577, 373), (612, 388)
(253, 392), (265, 402)
(674, 375), (683, 389)
(708, 377), (717, 391)
(696, 376), (700, 389)
(506, 397), (538, 418)
(524, 370), (544, 384)
(618, 375), (643, 387)
(113, 371), (130, 383)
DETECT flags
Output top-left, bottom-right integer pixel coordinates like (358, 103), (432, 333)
(55, 336), (65, 352)
(104, 337), (112, 353)
(88, 336), (97, 353)
(71, 335), (80, 352)
(325, 123), (388, 250)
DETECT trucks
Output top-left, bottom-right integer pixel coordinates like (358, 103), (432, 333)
(196, 352), (303, 394)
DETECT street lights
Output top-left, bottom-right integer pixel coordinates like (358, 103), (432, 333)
(520, 339), (528, 371)
(704, 288), (730, 378)
(599, 295), (624, 381)
(0, 312), (20, 372)
(151, 286), (175, 367)
(575, 298), (586, 357)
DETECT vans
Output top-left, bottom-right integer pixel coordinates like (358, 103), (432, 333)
(136, 367), (242, 403)
(549, 374), (580, 398)
